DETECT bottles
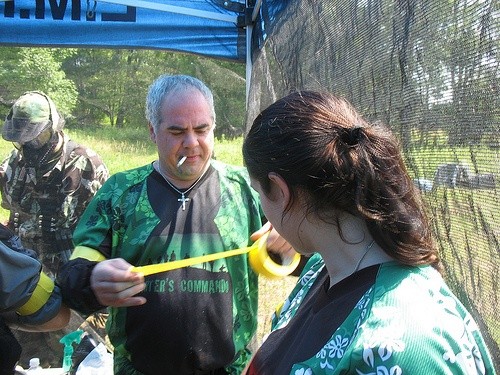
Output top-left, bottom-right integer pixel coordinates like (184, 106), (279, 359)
(26, 358), (43, 375)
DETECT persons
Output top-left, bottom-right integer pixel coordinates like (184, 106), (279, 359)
(0, 90), (111, 375)
(239, 89), (500, 375)
(55, 74), (316, 375)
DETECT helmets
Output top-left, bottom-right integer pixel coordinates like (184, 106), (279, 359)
(1, 91), (60, 168)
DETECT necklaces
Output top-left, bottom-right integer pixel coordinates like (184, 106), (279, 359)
(328, 239), (376, 290)
(157, 159), (211, 212)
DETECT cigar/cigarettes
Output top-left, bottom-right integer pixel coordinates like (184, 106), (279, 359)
(176, 156), (188, 168)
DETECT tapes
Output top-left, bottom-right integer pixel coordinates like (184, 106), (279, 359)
(133, 227), (301, 284)
